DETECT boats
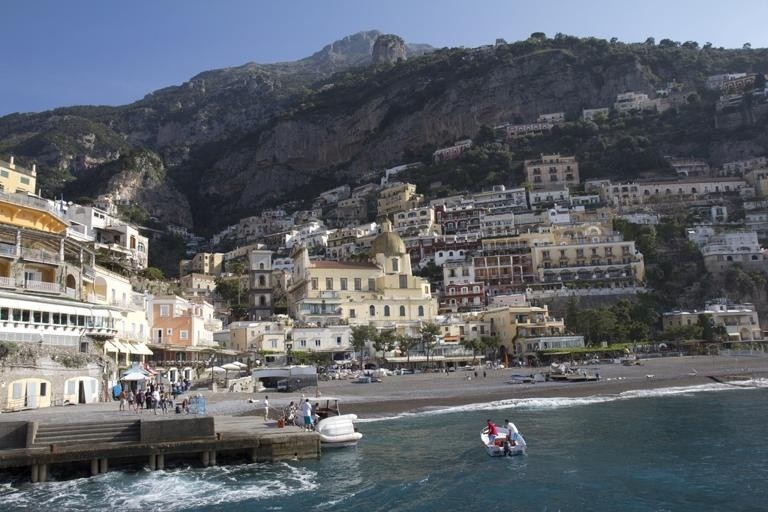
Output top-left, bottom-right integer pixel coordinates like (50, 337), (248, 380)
(297, 396), (365, 449)
(480, 423), (529, 457)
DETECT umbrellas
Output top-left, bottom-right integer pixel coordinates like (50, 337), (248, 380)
(231, 360), (247, 379)
(220, 363), (240, 379)
(204, 366), (225, 382)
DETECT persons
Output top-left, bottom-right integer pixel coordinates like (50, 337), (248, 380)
(583, 370), (589, 384)
(503, 418), (521, 446)
(312, 402), (320, 425)
(285, 401), (296, 427)
(482, 418), (497, 447)
(262, 395), (270, 423)
(116, 376), (196, 415)
(301, 398), (312, 432)
(595, 371), (599, 384)
